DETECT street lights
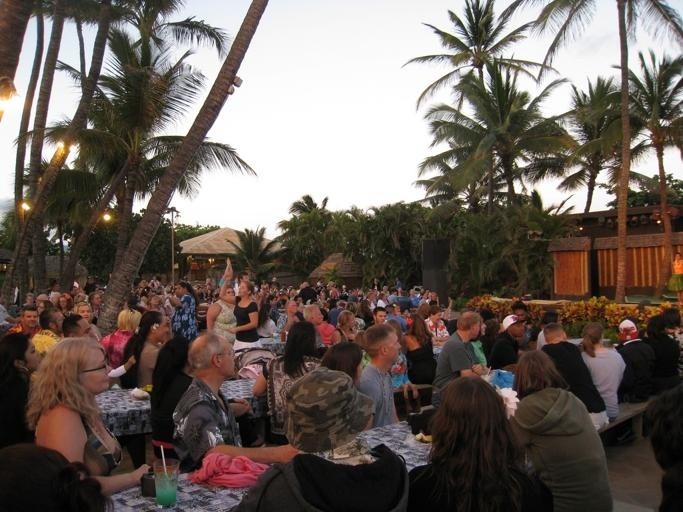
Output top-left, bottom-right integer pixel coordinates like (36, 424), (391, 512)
(21, 197), (35, 292)
(101, 212), (111, 285)
(166, 208), (179, 290)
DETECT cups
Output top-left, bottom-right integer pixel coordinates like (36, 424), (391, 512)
(142, 473), (156, 497)
(153, 458), (181, 507)
(280, 330), (287, 342)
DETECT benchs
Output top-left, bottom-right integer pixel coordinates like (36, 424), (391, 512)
(0, 281), (682, 512)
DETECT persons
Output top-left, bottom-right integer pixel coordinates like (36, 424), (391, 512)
(1, 258), (682, 510)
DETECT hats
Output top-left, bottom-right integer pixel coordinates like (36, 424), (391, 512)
(498, 315), (518, 333)
(283, 367), (374, 452)
(619, 320), (637, 334)
